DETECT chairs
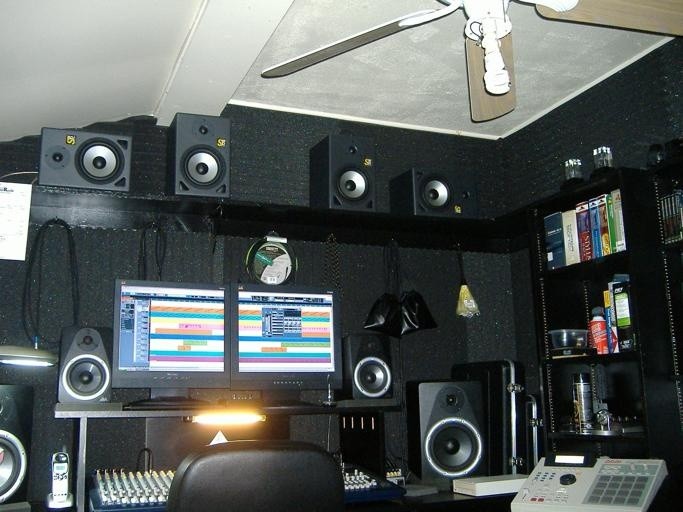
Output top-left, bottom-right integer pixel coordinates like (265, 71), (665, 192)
(168, 441), (344, 510)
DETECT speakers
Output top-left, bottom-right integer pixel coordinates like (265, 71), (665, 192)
(390, 167), (482, 217)
(406, 377), (494, 486)
(340, 330), (394, 399)
(1, 382), (35, 502)
(167, 113), (233, 199)
(39, 120), (134, 194)
(55, 326), (117, 405)
(308, 134), (380, 211)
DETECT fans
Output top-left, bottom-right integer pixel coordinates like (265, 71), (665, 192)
(261, 1), (683, 124)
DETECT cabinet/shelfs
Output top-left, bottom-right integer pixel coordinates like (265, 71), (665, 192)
(534, 165), (681, 460)
(54, 398), (475, 512)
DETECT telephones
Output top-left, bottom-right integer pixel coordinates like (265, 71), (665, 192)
(46, 445), (74, 508)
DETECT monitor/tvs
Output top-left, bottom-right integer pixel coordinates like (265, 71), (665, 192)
(236, 281), (339, 407)
(111, 273), (232, 411)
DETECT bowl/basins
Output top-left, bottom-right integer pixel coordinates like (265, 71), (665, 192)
(548, 329), (588, 348)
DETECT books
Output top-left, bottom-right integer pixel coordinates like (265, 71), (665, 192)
(604, 280), (637, 354)
(543, 189), (626, 271)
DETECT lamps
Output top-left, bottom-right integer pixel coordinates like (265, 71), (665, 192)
(463, 1), (511, 95)
(455, 240), (480, 317)
(0, 347), (59, 367)
